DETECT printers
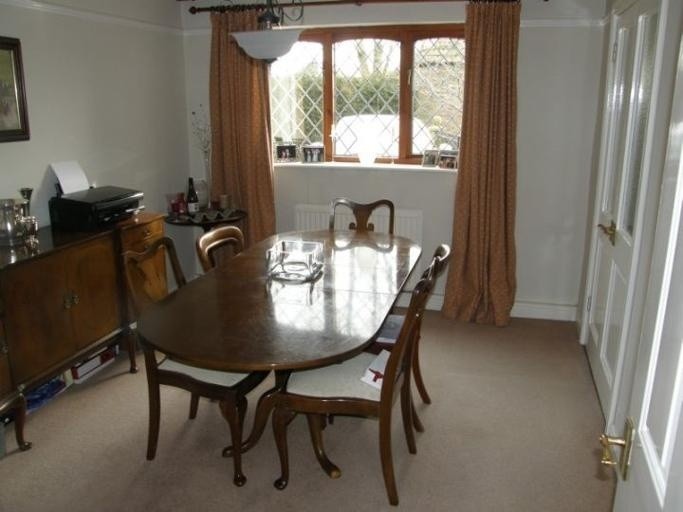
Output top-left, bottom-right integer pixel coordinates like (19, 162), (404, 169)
(47, 180), (147, 232)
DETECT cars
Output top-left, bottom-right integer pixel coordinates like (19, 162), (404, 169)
(310, 114), (452, 164)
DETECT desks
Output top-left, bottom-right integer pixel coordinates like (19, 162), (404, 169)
(163, 209), (248, 267)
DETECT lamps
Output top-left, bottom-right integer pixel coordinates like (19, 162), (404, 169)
(227, 0), (309, 65)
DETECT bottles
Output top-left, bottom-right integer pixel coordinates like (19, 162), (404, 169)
(165, 190), (187, 223)
(1, 187), (40, 249)
(186, 177), (200, 216)
(195, 180), (208, 208)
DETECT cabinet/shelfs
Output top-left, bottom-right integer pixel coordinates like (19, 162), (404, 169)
(577, 1), (683, 438)
(1, 214), (169, 450)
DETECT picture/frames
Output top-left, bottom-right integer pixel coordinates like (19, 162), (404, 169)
(0, 36), (30, 142)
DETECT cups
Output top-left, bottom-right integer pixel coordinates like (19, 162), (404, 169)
(220, 195), (229, 210)
